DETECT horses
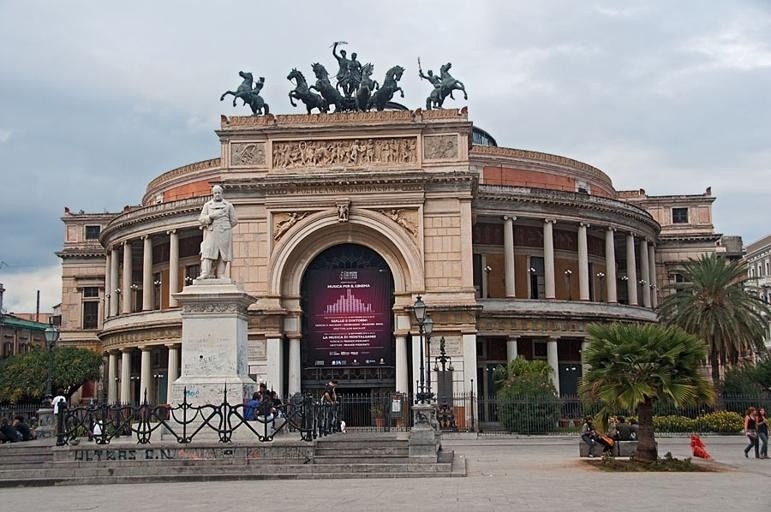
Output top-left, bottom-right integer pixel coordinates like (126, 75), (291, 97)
(308, 62), (344, 112)
(220, 71), (269, 116)
(366, 65), (406, 112)
(354, 62), (380, 112)
(426, 63), (467, 110)
(287, 67), (327, 114)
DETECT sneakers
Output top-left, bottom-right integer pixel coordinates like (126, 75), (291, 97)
(744, 450), (771, 459)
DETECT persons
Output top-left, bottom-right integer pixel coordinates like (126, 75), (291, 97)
(333, 41), (350, 98)
(581, 415), (606, 457)
(271, 139), (416, 163)
(0, 418), (19, 444)
(250, 76), (265, 94)
(757, 408), (770, 459)
(198, 184), (237, 281)
(630, 418), (640, 441)
(49, 387), (67, 436)
(743, 407), (760, 459)
(322, 377), (340, 406)
(274, 211), (308, 240)
(420, 69), (443, 101)
(12, 416), (32, 442)
(381, 209), (416, 236)
(244, 382), (283, 422)
(348, 52), (363, 96)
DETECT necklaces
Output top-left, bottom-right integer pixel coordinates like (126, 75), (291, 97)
(612, 417), (630, 441)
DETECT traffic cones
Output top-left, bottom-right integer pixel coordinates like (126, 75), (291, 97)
(691, 434), (711, 458)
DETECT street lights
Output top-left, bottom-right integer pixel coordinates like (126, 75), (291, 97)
(414, 293), (435, 404)
(41, 323), (60, 408)
(486, 368), (495, 399)
